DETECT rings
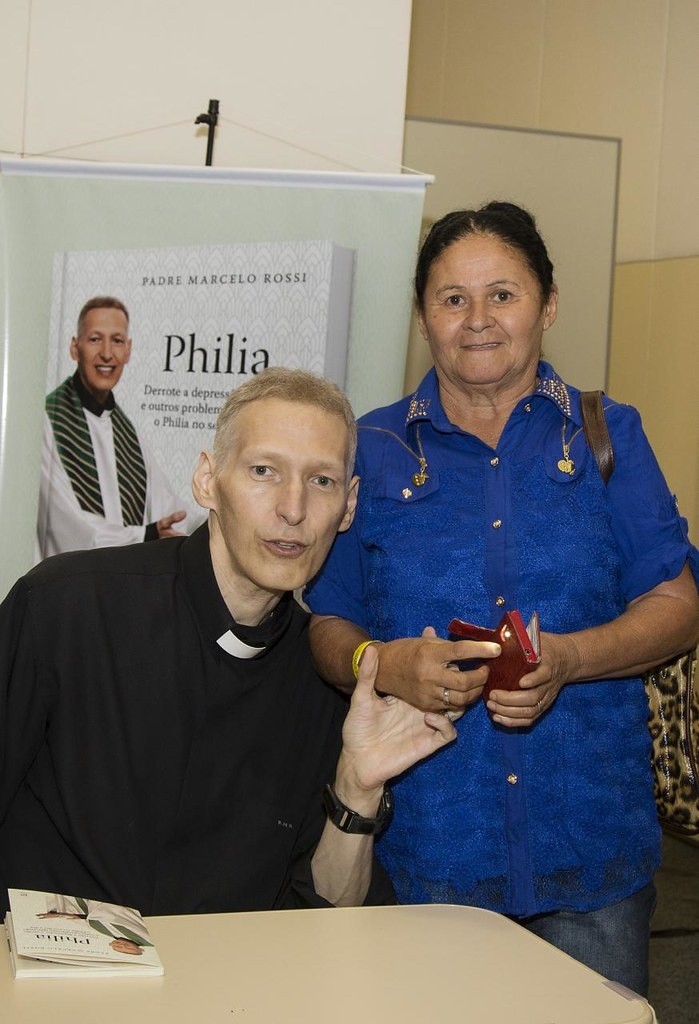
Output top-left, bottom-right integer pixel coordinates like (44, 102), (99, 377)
(537, 702), (543, 713)
(443, 688), (450, 704)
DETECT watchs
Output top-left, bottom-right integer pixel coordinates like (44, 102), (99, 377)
(322, 783), (395, 841)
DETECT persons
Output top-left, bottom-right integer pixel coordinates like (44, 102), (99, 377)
(33, 298), (187, 558)
(0, 367), (462, 948)
(36, 893), (154, 955)
(301, 201), (698, 999)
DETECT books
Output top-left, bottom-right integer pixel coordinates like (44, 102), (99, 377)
(49, 236), (352, 543)
(3, 887), (163, 979)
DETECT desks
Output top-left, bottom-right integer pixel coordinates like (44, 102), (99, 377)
(0, 903), (657, 1024)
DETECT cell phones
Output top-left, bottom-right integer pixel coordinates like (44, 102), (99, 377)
(477, 610), (542, 719)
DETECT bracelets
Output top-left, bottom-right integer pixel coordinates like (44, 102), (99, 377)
(351, 639), (385, 680)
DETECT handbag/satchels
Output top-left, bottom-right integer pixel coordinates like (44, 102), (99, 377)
(583, 388), (699, 844)
